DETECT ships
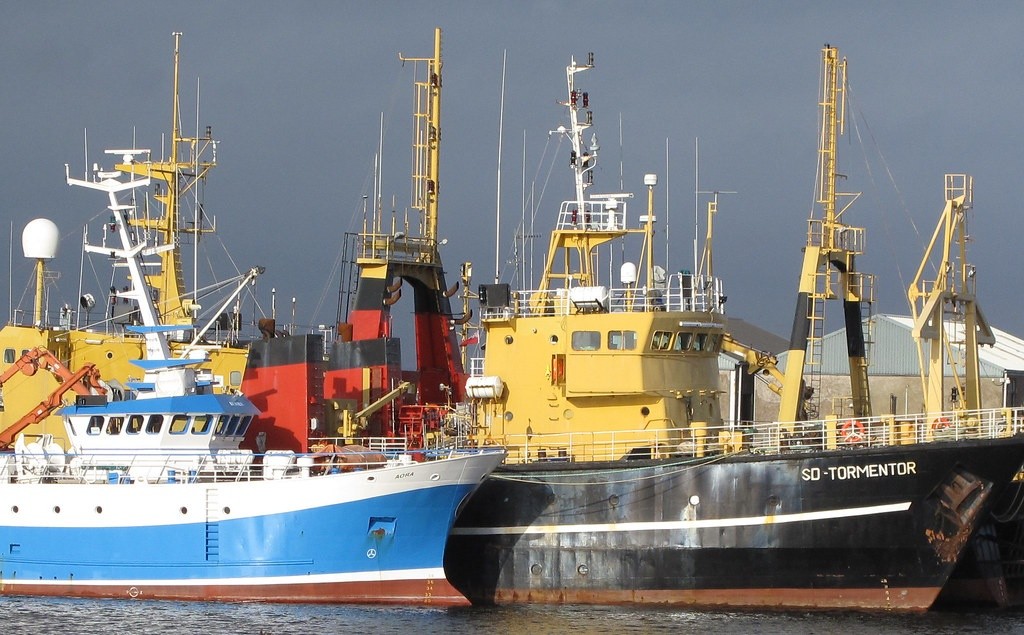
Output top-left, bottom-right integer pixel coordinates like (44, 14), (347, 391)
(0, 32), (507, 613)
(444, 39), (1023, 610)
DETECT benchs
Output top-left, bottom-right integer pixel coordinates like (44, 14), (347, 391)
(212, 450), (255, 481)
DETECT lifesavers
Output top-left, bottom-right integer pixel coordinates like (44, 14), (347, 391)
(932, 418), (950, 434)
(841, 420), (865, 442)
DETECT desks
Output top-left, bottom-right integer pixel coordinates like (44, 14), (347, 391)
(78, 464), (129, 485)
